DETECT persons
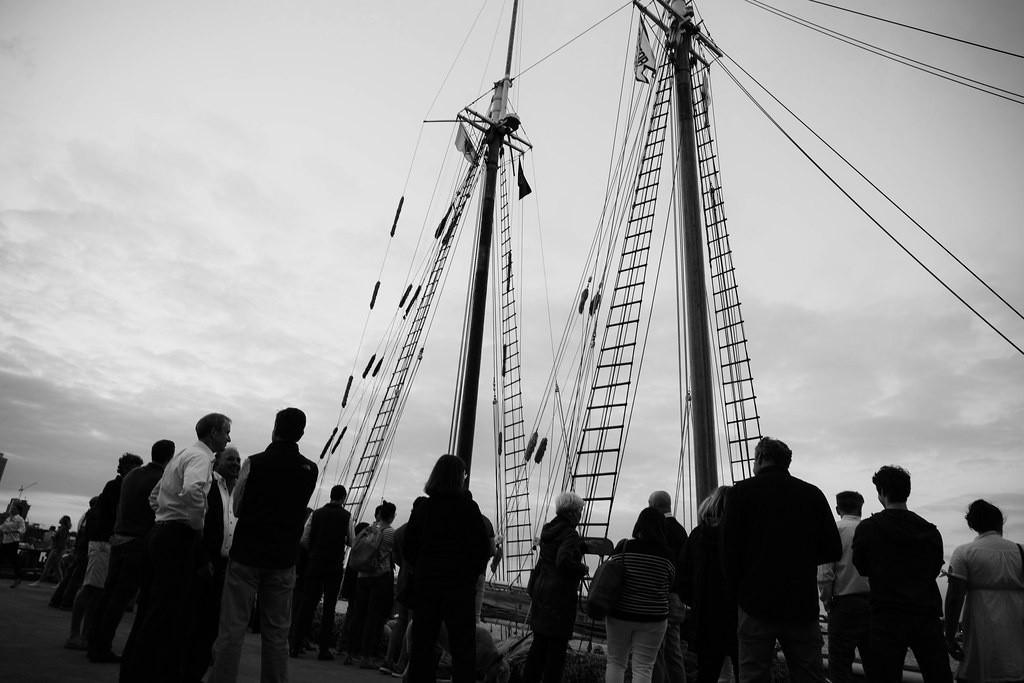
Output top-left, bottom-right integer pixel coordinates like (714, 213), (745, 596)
(0, 407), (496, 683)
(521, 493), (612, 683)
(607, 437), (1024, 683)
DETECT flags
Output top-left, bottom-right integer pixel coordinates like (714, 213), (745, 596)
(634, 18), (656, 84)
(700, 76), (711, 110)
(455, 125), (477, 164)
(518, 161), (532, 200)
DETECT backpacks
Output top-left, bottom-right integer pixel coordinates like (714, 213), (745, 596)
(349, 522), (392, 571)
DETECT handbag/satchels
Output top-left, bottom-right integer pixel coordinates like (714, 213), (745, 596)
(587, 539), (628, 620)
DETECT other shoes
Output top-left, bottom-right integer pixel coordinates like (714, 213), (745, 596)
(359, 655), (379, 669)
(64, 636), (87, 650)
(290, 647), (299, 658)
(10, 578), (21, 587)
(86, 648), (121, 662)
(392, 663), (405, 677)
(318, 649), (335, 660)
(28, 581), (41, 587)
(380, 660), (393, 673)
(51, 582), (60, 589)
(60, 603), (73, 610)
(49, 599), (60, 608)
(343, 653), (352, 665)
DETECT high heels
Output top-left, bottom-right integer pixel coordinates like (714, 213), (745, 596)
(299, 649), (306, 654)
(301, 641), (316, 651)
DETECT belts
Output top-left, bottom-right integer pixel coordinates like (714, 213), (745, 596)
(156, 521), (172, 526)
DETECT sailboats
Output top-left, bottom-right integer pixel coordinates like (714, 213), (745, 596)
(287, 0), (1024, 683)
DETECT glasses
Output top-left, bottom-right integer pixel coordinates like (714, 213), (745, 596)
(464, 473), (468, 478)
